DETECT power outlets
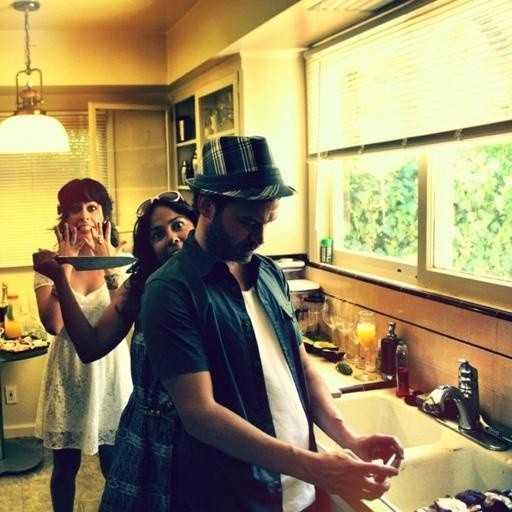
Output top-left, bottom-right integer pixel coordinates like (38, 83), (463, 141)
(5, 385), (17, 404)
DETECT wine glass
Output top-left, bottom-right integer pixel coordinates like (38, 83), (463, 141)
(321, 295), (378, 384)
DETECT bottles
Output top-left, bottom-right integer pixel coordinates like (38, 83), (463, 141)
(381, 321), (409, 396)
(321, 240), (333, 262)
(181, 159), (191, 185)
(0, 282), (23, 339)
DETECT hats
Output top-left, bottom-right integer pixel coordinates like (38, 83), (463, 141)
(185, 135), (296, 201)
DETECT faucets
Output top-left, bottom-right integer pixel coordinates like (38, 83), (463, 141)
(421, 359), (480, 434)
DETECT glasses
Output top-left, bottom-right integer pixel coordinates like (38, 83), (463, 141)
(136, 191), (190, 219)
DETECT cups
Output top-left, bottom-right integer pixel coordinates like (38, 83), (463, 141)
(178, 115), (191, 141)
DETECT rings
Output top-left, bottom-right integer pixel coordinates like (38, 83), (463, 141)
(96, 235), (106, 245)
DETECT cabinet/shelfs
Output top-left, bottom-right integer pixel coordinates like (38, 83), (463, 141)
(87, 56), (307, 257)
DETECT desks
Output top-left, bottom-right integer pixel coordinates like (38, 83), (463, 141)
(0, 333), (48, 476)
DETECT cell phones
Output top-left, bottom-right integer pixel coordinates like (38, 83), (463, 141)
(373, 450), (399, 484)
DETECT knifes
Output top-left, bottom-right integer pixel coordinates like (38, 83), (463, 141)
(58, 256), (137, 272)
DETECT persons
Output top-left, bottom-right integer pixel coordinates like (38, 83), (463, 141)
(139, 134), (404, 512)
(32, 176), (132, 512)
(33, 191), (198, 512)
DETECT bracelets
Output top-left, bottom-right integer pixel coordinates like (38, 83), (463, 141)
(105, 274), (118, 289)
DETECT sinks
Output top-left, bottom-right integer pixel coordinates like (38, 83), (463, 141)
(362, 442), (511, 511)
(313, 389), (458, 466)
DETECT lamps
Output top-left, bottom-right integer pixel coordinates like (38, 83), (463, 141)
(0, 0), (71, 155)
